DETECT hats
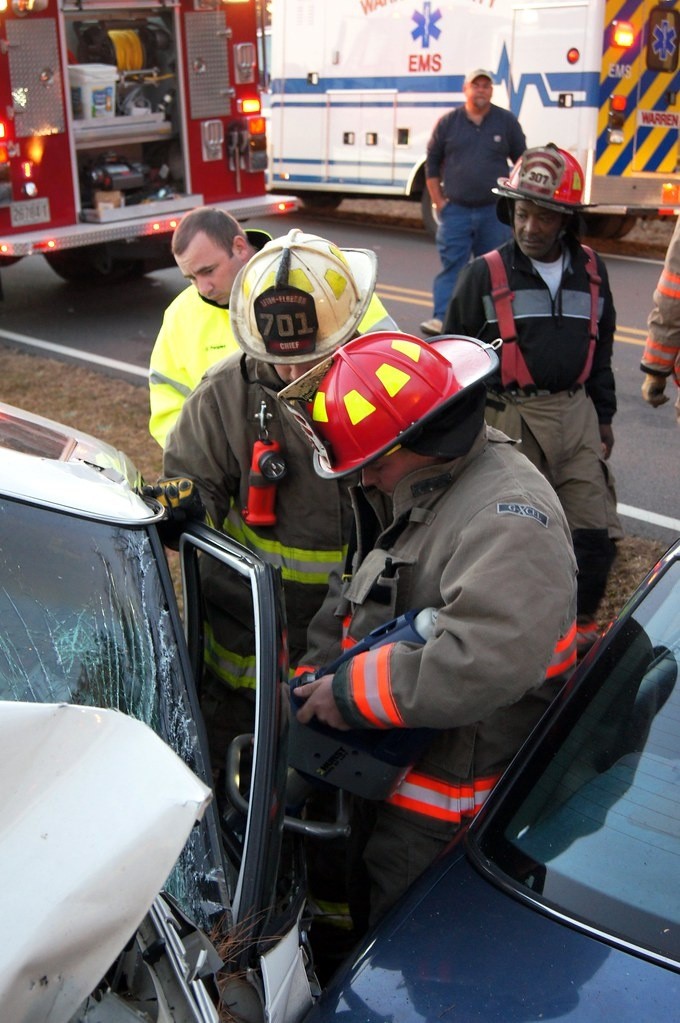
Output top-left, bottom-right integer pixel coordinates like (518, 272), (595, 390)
(465, 68), (494, 85)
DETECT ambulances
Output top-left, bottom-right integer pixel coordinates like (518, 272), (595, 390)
(257, 1), (679, 239)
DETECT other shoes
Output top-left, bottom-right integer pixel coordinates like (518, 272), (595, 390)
(420, 321), (444, 335)
(576, 623), (597, 653)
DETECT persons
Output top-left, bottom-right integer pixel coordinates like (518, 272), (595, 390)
(293, 335), (579, 943)
(641, 214), (680, 421)
(420, 69), (526, 336)
(147, 207), (402, 455)
(138, 229), (378, 973)
(442, 144), (622, 648)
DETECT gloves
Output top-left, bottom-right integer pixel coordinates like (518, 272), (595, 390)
(641, 373), (670, 408)
(142, 479), (206, 526)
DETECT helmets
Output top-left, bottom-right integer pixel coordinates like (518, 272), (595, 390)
(310, 329), (500, 480)
(228, 223), (379, 363)
(489, 141), (598, 214)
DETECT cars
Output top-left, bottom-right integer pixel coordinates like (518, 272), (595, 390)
(296, 538), (680, 1020)
(0, 396), (326, 1022)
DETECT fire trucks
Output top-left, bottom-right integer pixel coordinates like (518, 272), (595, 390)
(0, 2), (303, 286)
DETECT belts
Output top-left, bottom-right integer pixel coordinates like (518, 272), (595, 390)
(449, 198), (498, 209)
